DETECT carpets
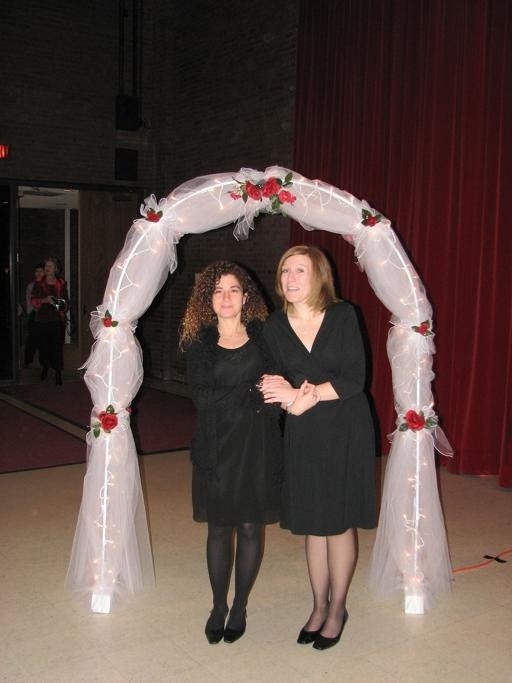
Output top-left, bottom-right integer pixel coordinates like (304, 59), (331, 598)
(0, 376), (199, 475)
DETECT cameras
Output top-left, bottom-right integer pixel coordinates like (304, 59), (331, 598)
(51, 295), (66, 308)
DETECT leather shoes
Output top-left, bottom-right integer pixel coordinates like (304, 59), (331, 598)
(205, 606), (228, 643)
(312, 609), (348, 650)
(298, 616), (323, 644)
(224, 605), (246, 641)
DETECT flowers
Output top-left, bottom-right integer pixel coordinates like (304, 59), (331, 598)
(223, 170), (301, 212)
(143, 206), (164, 223)
(396, 408), (440, 433)
(358, 207), (382, 227)
(99, 308), (121, 330)
(410, 318), (433, 338)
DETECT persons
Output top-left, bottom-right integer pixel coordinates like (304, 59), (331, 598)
(257, 243), (381, 651)
(175, 259), (287, 646)
(23, 262), (46, 366)
(30, 256), (71, 386)
